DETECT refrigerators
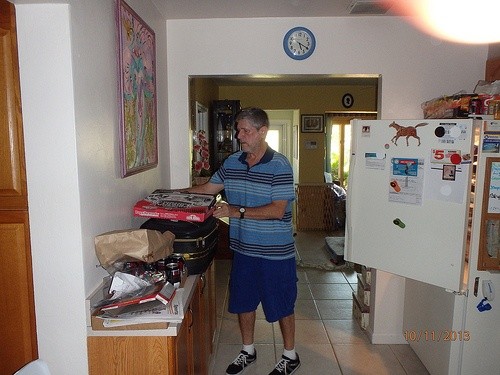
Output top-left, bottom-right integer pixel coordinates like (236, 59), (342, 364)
(343, 118), (500, 375)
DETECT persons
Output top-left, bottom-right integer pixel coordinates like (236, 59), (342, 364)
(181, 107), (301, 375)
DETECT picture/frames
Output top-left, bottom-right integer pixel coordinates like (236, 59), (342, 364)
(302, 114), (327, 134)
(114, 0), (158, 179)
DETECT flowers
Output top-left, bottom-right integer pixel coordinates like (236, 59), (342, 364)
(193, 129), (211, 177)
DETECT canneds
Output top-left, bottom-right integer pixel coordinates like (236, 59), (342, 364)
(157, 254), (185, 288)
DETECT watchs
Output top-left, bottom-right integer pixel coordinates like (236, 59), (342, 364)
(239, 208), (246, 219)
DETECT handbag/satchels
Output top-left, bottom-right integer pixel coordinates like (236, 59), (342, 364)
(94, 227), (176, 270)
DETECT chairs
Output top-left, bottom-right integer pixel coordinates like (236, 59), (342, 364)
(325, 184), (346, 234)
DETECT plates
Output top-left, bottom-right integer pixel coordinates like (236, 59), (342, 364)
(342, 93), (353, 107)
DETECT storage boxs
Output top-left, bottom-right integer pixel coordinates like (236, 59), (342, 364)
(352, 265), (371, 330)
(99, 282), (176, 317)
(133, 189), (216, 222)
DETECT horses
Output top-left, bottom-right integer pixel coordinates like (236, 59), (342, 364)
(388, 121), (429, 147)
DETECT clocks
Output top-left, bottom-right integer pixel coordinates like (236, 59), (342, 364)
(283, 26), (316, 60)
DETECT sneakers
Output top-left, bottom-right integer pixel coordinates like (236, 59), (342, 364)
(267, 351), (301, 375)
(225, 347), (257, 375)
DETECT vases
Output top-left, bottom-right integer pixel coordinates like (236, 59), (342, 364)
(195, 177), (210, 186)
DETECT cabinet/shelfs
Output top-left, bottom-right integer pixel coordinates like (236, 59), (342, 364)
(88, 256), (217, 375)
(213, 100), (241, 174)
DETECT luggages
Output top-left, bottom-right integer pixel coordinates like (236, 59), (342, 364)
(140, 215), (220, 275)
